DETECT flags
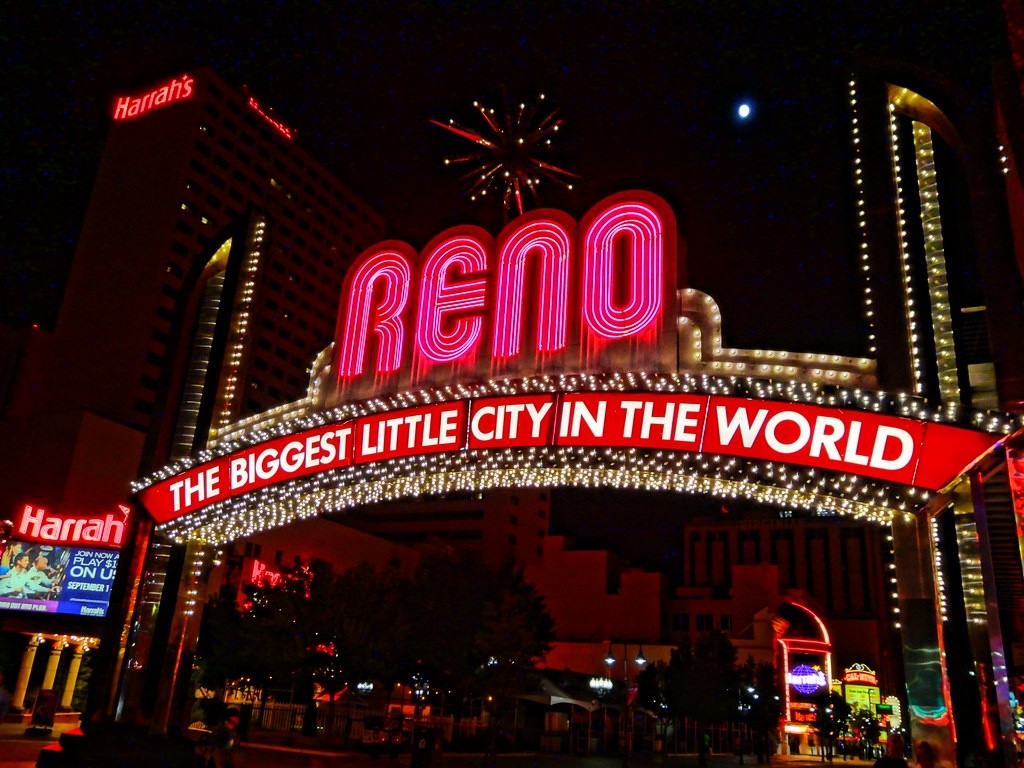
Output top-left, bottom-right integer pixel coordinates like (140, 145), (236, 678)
(889, 85), (966, 151)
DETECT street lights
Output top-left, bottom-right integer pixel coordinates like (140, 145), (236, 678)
(604, 634), (647, 768)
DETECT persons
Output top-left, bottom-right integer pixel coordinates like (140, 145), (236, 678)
(872, 732), (953, 768)
(704, 733), (711, 760)
(190, 730), (235, 768)
